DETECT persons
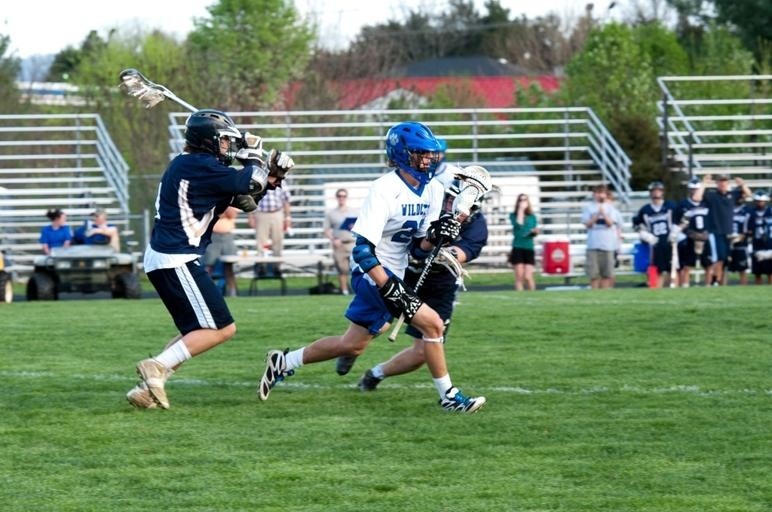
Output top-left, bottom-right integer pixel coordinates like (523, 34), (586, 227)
(433, 133), (467, 188)
(579, 171), (772, 290)
(82, 206), (121, 255)
(205, 204), (241, 299)
(255, 120), (487, 413)
(40, 206), (76, 255)
(508, 192), (539, 292)
(247, 177), (293, 278)
(124, 108), (296, 413)
(334, 163), (492, 393)
(324, 187), (359, 294)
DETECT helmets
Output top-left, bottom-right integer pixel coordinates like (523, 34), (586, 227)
(184, 109), (243, 167)
(648, 182), (664, 191)
(686, 178), (700, 189)
(752, 189), (772, 201)
(385, 121), (440, 184)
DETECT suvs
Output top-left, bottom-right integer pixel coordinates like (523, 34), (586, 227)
(27, 229), (141, 301)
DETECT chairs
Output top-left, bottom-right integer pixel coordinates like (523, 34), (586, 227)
(246, 262), (286, 298)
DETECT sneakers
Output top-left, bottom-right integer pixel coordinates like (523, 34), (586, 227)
(335, 353), (359, 376)
(437, 386), (486, 413)
(135, 357), (175, 409)
(256, 347), (294, 401)
(126, 380), (158, 409)
(357, 369), (383, 392)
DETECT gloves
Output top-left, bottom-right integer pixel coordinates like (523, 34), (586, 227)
(235, 132), (265, 167)
(376, 275), (413, 316)
(427, 213), (461, 247)
(261, 147), (294, 180)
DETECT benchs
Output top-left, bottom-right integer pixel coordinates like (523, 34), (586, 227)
(533, 234), (641, 286)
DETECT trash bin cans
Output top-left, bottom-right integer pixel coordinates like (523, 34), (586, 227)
(543, 233), (570, 274)
